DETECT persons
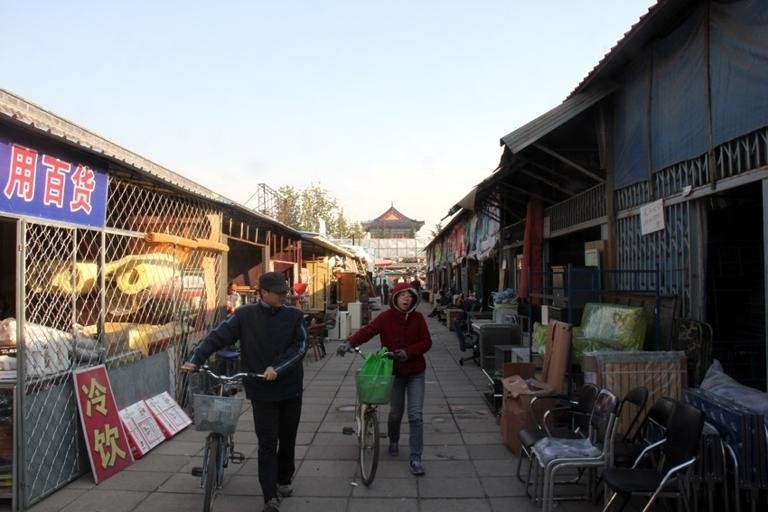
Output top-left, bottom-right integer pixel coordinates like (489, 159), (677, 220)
(383, 279), (390, 305)
(357, 276), (370, 325)
(179, 273), (308, 512)
(227, 280), (243, 310)
(391, 273), (429, 292)
(427, 291), (448, 317)
(335, 282), (433, 477)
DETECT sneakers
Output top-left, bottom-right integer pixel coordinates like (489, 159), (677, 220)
(409, 460), (424, 475)
(263, 498), (281, 512)
(279, 484), (293, 497)
(389, 442), (398, 456)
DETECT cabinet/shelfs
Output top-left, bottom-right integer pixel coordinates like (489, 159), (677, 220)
(548, 306), (568, 323)
(551, 265), (567, 308)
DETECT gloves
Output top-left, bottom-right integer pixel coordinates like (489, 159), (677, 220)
(337, 343), (349, 357)
(395, 349), (408, 361)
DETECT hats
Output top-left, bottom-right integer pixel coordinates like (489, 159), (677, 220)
(259, 272), (292, 294)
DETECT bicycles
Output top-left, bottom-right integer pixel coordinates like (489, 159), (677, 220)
(336, 344), (405, 487)
(181, 363), (267, 512)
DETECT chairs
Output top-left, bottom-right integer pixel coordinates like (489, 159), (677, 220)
(515, 383), (741, 512)
(309, 317), (335, 361)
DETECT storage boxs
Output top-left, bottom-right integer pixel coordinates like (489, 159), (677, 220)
(499, 375), (556, 444)
